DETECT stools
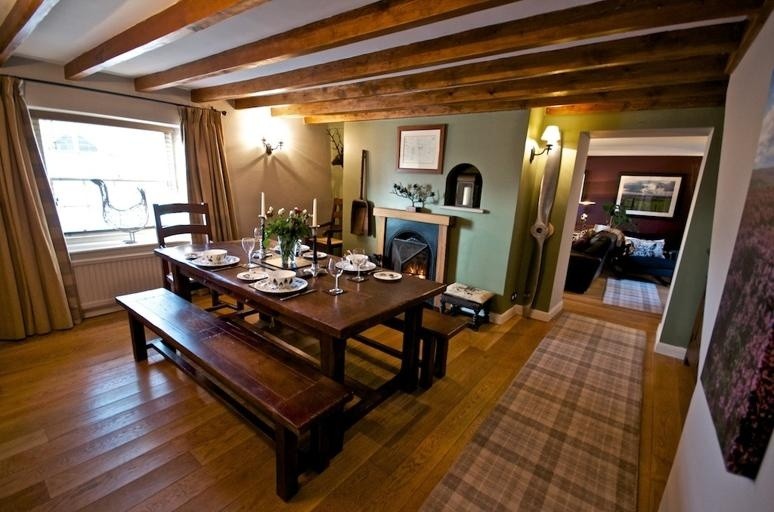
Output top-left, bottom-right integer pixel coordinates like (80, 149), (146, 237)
(440, 283), (497, 332)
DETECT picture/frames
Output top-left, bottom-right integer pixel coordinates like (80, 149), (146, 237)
(610, 172), (687, 221)
(396, 124), (446, 174)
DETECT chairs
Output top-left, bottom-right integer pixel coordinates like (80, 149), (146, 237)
(153, 203), (244, 320)
(305, 198), (343, 257)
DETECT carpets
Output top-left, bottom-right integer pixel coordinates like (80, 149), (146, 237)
(418, 312), (647, 512)
(603, 277), (663, 315)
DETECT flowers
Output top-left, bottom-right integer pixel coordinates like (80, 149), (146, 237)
(391, 184), (435, 206)
(262, 207), (313, 261)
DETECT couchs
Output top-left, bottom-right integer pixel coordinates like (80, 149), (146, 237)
(607, 231), (674, 287)
(565, 233), (617, 293)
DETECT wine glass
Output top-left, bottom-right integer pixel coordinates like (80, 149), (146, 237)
(254, 228), (266, 255)
(328, 257), (344, 295)
(347, 248), (366, 281)
(242, 237), (256, 268)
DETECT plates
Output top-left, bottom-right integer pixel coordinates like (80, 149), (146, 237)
(336, 260), (375, 272)
(274, 244), (310, 253)
(302, 250), (327, 259)
(255, 276), (308, 294)
(373, 271), (402, 281)
(191, 256), (241, 267)
(237, 272), (267, 281)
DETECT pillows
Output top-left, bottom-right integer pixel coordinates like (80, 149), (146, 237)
(622, 236), (665, 259)
(572, 232), (591, 252)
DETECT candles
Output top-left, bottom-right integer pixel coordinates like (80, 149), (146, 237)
(261, 192), (264, 215)
(312, 199), (317, 226)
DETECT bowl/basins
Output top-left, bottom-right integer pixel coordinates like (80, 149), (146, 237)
(268, 269), (296, 288)
(201, 250), (227, 264)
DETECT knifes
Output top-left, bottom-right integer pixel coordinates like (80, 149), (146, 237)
(210, 264), (242, 272)
(280, 289), (316, 301)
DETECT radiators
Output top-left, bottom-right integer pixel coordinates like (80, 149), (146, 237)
(72, 252), (171, 311)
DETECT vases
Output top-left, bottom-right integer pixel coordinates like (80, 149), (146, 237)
(277, 235), (298, 265)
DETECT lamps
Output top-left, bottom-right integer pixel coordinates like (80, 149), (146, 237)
(579, 201), (596, 230)
(530, 126), (560, 163)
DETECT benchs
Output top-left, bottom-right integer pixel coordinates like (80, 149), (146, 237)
(351, 308), (469, 390)
(116, 288), (353, 501)
